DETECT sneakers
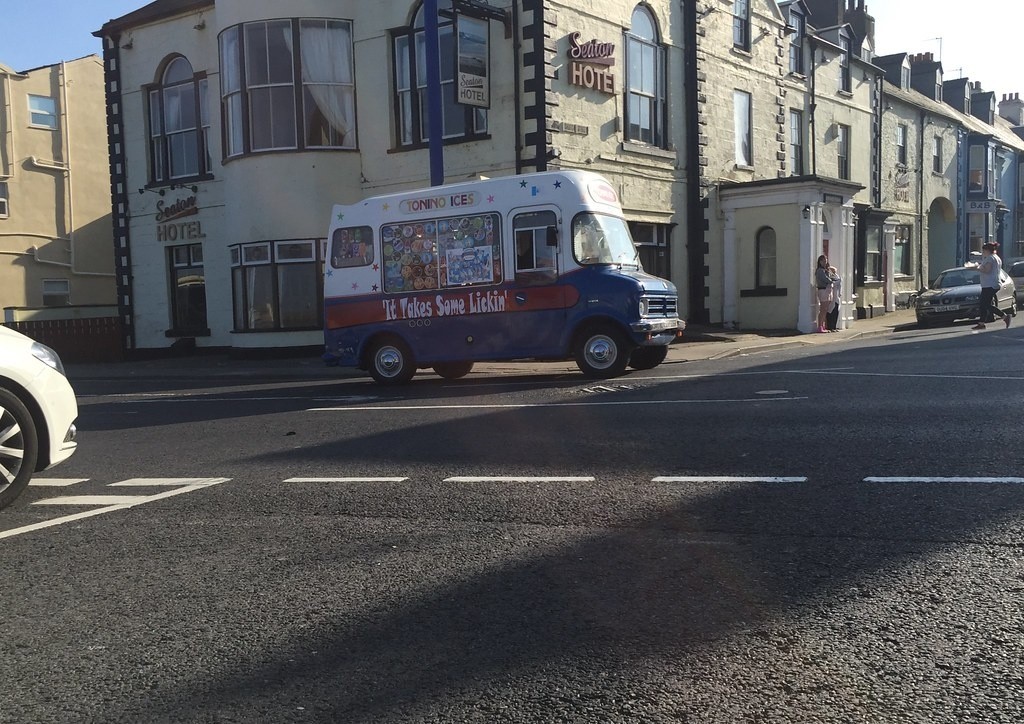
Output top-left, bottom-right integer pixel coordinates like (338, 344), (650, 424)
(971, 323), (985, 329)
(1003, 314), (1012, 328)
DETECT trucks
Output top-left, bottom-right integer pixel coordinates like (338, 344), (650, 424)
(323, 169), (684, 387)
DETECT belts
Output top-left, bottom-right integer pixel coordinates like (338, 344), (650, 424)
(818, 287), (826, 289)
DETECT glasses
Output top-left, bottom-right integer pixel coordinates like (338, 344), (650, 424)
(983, 243), (989, 247)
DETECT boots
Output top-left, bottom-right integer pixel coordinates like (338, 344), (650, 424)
(818, 325), (831, 332)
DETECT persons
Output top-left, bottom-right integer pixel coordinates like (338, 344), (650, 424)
(815, 255), (842, 333)
(971, 243), (1011, 329)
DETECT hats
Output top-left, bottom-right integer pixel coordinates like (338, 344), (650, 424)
(990, 242), (1000, 253)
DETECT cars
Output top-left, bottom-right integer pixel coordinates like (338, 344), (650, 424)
(0, 325), (78, 512)
(916, 266), (1016, 327)
(1007, 261), (1024, 308)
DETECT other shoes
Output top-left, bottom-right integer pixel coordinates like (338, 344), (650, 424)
(832, 329), (839, 332)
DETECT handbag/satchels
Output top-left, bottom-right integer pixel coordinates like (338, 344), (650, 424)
(825, 284), (831, 294)
